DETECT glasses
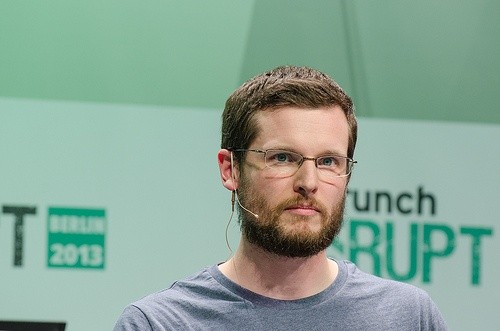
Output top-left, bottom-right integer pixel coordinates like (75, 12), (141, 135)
(229, 147), (356, 178)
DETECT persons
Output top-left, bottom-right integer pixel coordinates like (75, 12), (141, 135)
(111, 63), (451, 330)
